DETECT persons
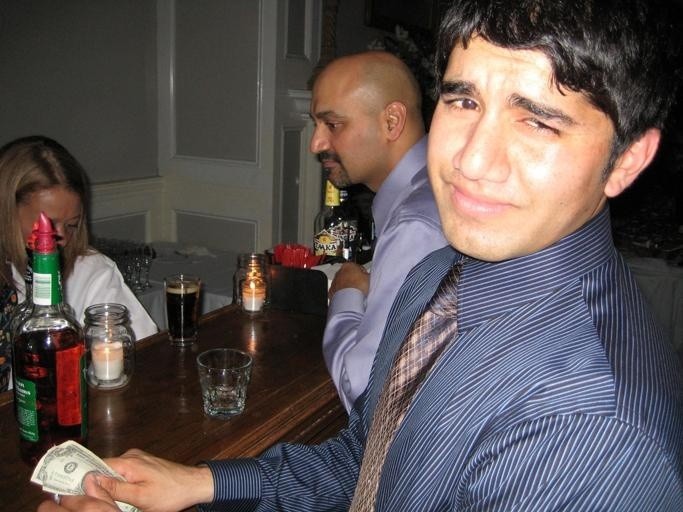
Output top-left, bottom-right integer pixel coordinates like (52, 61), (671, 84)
(308, 50), (450, 417)
(0, 135), (161, 391)
(37, 0), (683, 512)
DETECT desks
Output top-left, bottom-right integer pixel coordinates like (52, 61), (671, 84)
(2, 270), (344, 512)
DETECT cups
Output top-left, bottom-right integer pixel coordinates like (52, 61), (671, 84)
(196, 346), (253, 418)
(84, 303), (137, 391)
(161, 274), (202, 349)
(234, 250), (274, 322)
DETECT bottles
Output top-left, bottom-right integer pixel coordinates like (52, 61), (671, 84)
(12, 210), (90, 468)
(313, 183), (361, 265)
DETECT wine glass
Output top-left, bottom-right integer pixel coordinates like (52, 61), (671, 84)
(113, 256), (154, 294)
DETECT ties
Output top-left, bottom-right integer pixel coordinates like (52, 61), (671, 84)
(350, 261), (463, 507)
